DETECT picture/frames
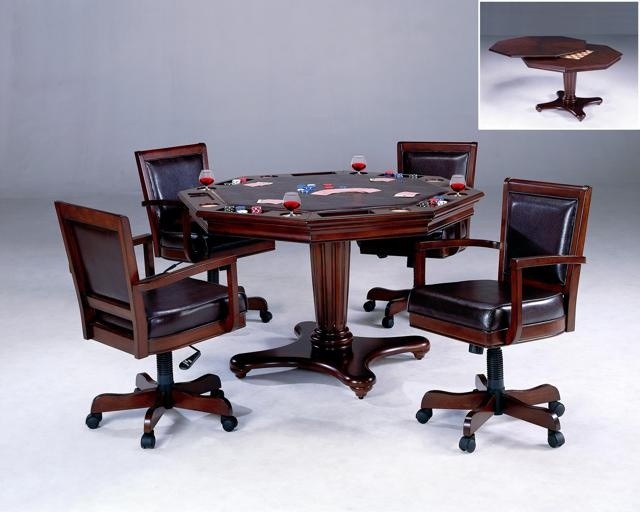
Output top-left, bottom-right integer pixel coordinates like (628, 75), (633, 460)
(476, 2), (639, 132)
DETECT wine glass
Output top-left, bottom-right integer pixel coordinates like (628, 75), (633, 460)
(449, 175), (467, 197)
(281, 191), (302, 218)
(199, 169), (215, 192)
(351, 155), (367, 177)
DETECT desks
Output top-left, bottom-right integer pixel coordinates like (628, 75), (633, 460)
(488, 35), (623, 123)
(173, 168), (485, 401)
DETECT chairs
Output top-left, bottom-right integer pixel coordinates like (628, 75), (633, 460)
(51, 196), (243, 452)
(130, 142), (277, 326)
(403, 175), (591, 453)
(356, 143), (480, 335)
